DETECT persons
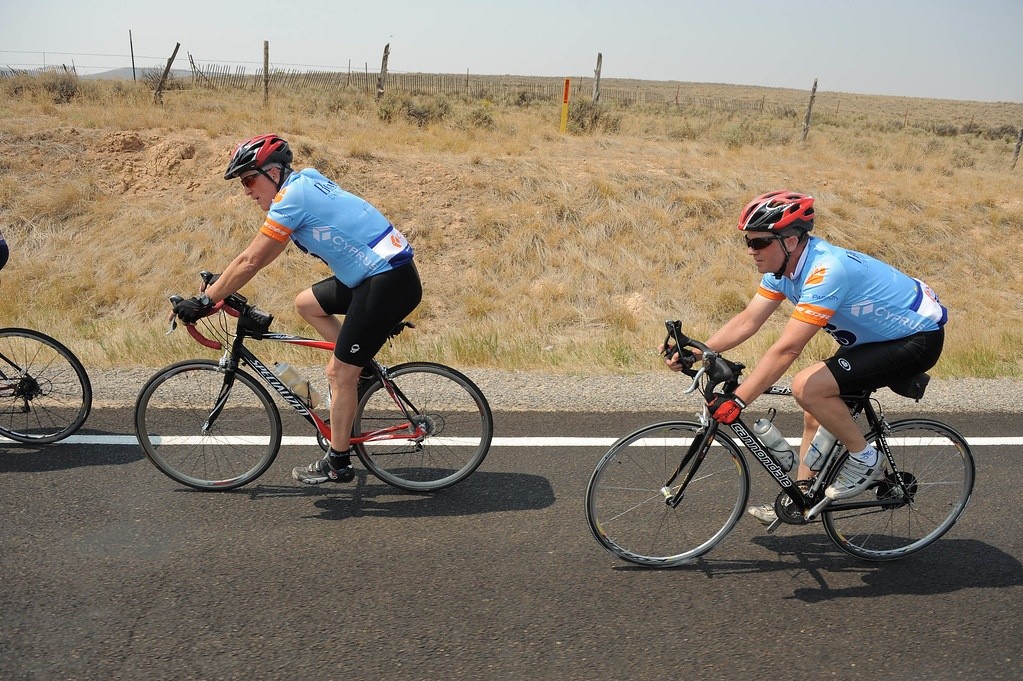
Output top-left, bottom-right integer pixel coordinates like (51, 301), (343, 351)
(0, 229), (10, 271)
(658, 189), (950, 524)
(165, 130), (424, 483)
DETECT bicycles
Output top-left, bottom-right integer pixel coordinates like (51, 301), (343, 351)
(585, 320), (976, 568)
(0, 326), (92, 444)
(133, 271), (493, 494)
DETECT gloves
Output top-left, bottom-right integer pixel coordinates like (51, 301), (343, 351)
(705, 392), (747, 426)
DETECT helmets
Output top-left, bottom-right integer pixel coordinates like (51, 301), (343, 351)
(224, 133), (293, 180)
(738, 190), (815, 231)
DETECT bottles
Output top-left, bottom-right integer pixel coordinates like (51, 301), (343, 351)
(325, 389), (332, 410)
(803, 424), (837, 470)
(275, 362), (322, 408)
(754, 418), (800, 471)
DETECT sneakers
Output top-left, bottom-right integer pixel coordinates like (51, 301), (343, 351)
(357, 372), (379, 405)
(747, 502), (778, 521)
(825, 446), (886, 500)
(292, 453), (354, 486)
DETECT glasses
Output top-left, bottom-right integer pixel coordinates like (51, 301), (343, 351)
(240, 168), (271, 188)
(745, 233), (791, 249)
